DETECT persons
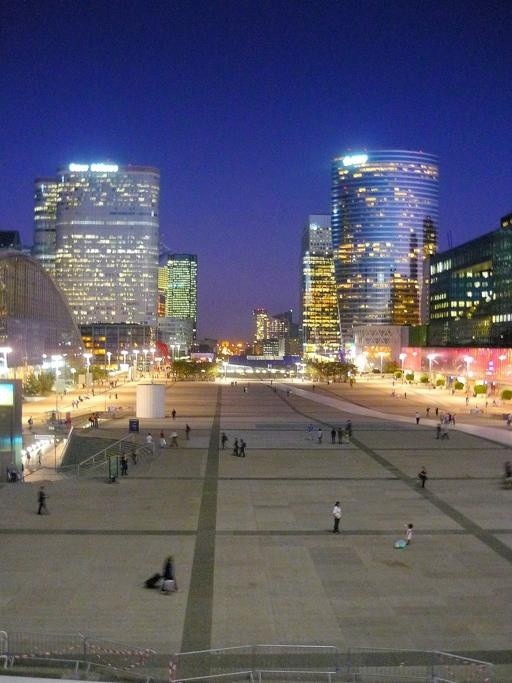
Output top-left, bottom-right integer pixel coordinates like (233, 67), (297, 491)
(416, 407), (456, 440)
(232, 375), (413, 400)
(159, 553), (182, 594)
(6, 463), (24, 482)
(330, 500), (344, 534)
(419, 469), (427, 487)
(504, 462), (512, 486)
(63, 379), (119, 428)
(221, 433), (246, 457)
(452, 387), (512, 425)
(405, 522), (414, 546)
(307, 419), (353, 444)
(36, 483), (50, 515)
(120, 452), (128, 476)
(146, 408), (191, 453)
(28, 416), (33, 429)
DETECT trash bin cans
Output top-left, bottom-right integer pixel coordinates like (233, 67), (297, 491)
(129, 419), (139, 432)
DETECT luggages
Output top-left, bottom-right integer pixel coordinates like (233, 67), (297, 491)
(146, 572), (165, 586)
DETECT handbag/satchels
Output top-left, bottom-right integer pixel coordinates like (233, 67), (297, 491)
(164, 580), (176, 592)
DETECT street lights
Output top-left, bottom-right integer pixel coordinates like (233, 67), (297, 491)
(347, 344), (511, 402)
(49, 343), (182, 392)
(0, 345), (14, 380)
